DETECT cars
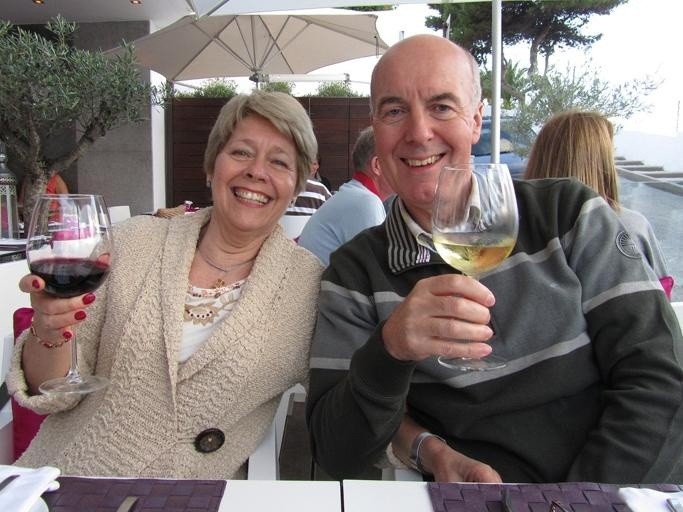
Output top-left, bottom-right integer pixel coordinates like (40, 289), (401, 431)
(471, 115), (542, 175)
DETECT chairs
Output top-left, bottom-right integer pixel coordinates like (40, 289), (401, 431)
(0, 308), (421, 481)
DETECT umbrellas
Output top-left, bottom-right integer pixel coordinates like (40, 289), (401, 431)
(92, 7), (388, 85)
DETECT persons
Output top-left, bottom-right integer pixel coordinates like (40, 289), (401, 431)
(520, 109), (669, 283)
(1, 87), (502, 484)
(301, 33), (682, 484)
(281, 178), (334, 217)
(14, 155), (75, 224)
(307, 155), (332, 193)
(295, 122), (399, 271)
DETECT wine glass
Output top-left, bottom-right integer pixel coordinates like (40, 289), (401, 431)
(24, 193), (116, 398)
(430, 161), (521, 373)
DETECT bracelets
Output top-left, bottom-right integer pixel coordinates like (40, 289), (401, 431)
(408, 430), (446, 476)
(29, 317), (70, 349)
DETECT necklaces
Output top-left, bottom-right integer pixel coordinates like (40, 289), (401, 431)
(212, 278), (228, 291)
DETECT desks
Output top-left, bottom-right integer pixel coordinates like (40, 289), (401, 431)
(0, 464), (683, 512)
